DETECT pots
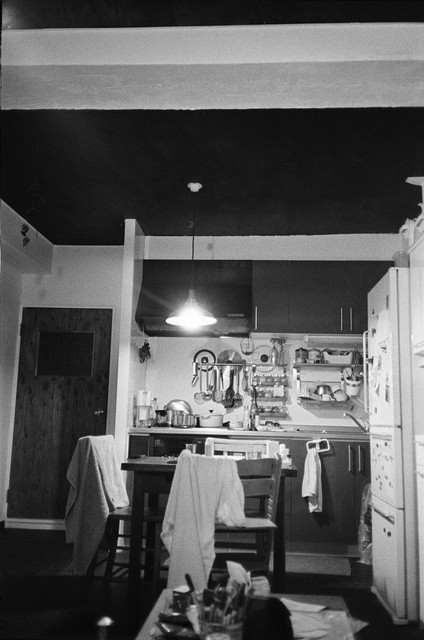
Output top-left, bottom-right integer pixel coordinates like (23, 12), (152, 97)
(197, 409), (224, 428)
(155, 409), (182, 426)
(172, 413), (196, 427)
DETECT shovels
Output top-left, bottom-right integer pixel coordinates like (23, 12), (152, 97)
(224, 370), (234, 409)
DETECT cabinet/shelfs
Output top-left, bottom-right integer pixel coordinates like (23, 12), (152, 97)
(290, 440), (371, 557)
(251, 333), (368, 432)
(252, 262), (289, 331)
(289, 261), (394, 333)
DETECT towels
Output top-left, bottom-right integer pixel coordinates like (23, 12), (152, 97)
(301, 449), (323, 514)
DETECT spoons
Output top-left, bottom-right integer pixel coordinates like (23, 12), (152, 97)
(203, 363), (213, 401)
(234, 370), (242, 401)
(194, 364), (204, 402)
(213, 366), (224, 402)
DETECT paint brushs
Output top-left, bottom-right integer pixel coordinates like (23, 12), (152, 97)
(184, 573), (247, 625)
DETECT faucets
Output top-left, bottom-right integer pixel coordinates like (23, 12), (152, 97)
(342, 412), (370, 434)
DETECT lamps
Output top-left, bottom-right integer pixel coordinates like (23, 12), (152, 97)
(164, 215), (218, 329)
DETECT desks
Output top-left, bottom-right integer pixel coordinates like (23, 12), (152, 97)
(121, 456), (298, 590)
(135, 588), (370, 639)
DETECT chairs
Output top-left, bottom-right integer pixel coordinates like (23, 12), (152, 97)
(65, 434), (165, 575)
(174, 450), (282, 589)
